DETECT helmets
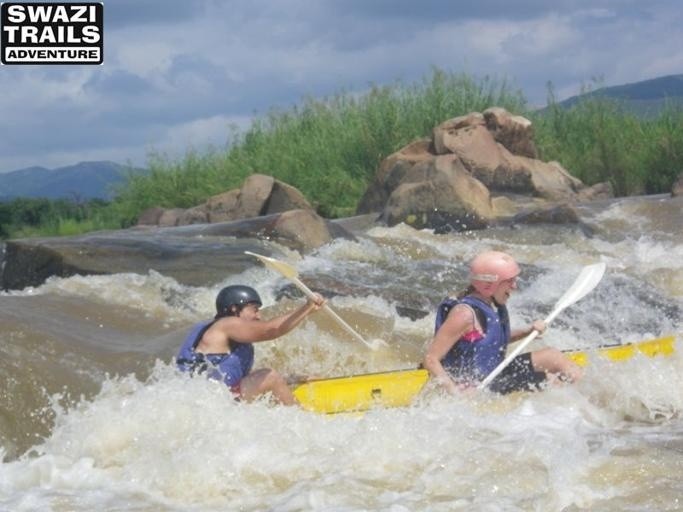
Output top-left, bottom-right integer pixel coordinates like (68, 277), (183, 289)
(471, 251), (520, 298)
(216, 286), (262, 316)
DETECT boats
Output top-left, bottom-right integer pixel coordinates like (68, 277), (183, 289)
(282, 336), (677, 414)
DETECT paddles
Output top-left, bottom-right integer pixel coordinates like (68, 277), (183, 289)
(244, 249), (374, 350)
(479, 264), (606, 387)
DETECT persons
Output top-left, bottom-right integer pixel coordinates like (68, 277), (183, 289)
(421, 250), (579, 399)
(171, 285), (328, 407)
